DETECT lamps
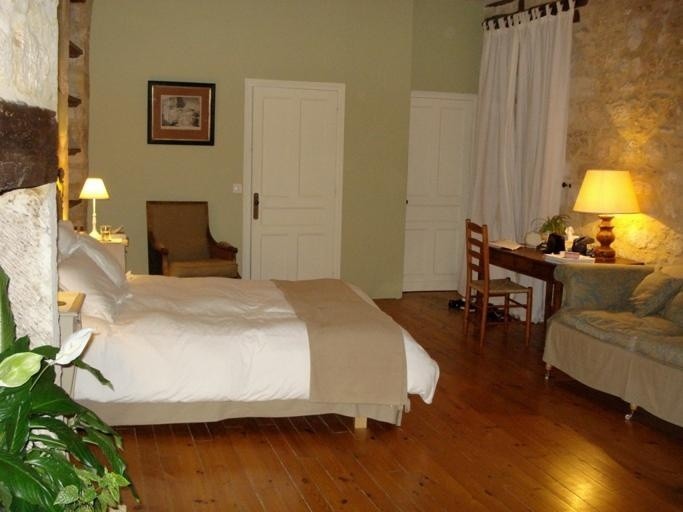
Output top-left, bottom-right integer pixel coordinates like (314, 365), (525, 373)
(572, 169), (642, 264)
(77, 175), (111, 241)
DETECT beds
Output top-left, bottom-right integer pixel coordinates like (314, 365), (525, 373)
(57, 267), (442, 433)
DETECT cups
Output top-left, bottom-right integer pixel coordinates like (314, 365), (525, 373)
(99, 225), (111, 240)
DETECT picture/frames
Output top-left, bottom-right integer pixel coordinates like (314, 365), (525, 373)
(145, 80), (217, 145)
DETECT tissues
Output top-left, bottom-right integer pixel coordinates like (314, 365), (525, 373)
(563, 225), (580, 250)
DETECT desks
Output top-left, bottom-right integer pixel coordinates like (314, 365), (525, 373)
(488, 239), (646, 335)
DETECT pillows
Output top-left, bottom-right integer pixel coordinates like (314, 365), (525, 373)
(628, 269), (683, 318)
(56, 219), (129, 325)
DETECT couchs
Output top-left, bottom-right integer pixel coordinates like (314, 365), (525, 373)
(543, 262), (683, 429)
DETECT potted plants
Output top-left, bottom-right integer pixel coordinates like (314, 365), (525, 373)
(531, 212), (574, 254)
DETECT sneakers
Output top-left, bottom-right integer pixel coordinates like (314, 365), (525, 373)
(487, 311), (512, 321)
(449, 299), (476, 312)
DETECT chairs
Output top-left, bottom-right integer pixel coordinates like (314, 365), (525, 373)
(462, 217), (533, 354)
(144, 198), (241, 279)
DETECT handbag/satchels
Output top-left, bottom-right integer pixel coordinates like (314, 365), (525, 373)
(546, 233), (566, 253)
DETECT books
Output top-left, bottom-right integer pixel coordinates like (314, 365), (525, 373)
(487, 240), (501, 248)
(542, 251), (595, 265)
(494, 239), (521, 251)
(55, 291), (86, 317)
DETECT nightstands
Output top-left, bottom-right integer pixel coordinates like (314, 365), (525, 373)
(57, 287), (87, 397)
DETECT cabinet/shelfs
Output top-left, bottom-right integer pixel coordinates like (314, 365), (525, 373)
(66, 38), (83, 209)
